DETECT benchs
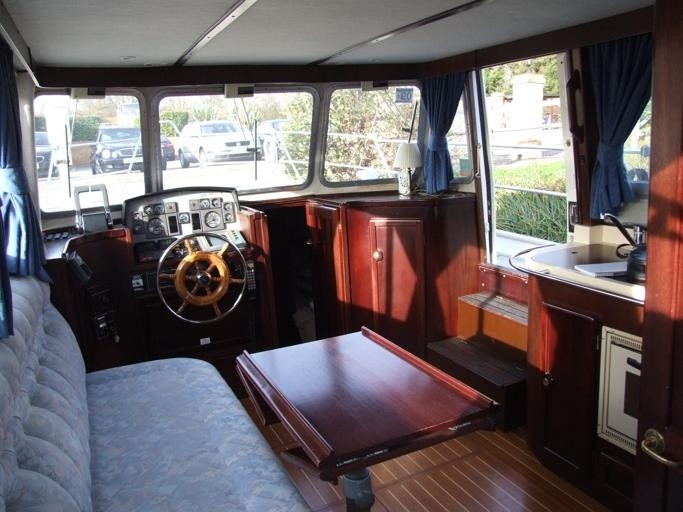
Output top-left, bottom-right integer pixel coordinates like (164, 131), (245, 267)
(1, 275), (317, 510)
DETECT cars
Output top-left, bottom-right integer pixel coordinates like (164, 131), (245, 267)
(35, 132), (57, 176)
(258, 119), (295, 159)
(90, 125), (166, 174)
(162, 134), (175, 162)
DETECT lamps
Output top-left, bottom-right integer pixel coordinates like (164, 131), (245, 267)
(392, 141), (423, 195)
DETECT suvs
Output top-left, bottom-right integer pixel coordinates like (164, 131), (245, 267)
(178, 120), (262, 168)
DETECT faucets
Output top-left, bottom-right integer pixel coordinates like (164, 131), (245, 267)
(599, 211), (635, 246)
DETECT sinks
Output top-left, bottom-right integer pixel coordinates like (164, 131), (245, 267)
(575, 261), (627, 277)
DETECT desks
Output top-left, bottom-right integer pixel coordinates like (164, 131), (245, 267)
(234, 322), (501, 510)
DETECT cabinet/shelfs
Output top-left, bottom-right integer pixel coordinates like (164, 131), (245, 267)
(303, 188), (480, 359)
